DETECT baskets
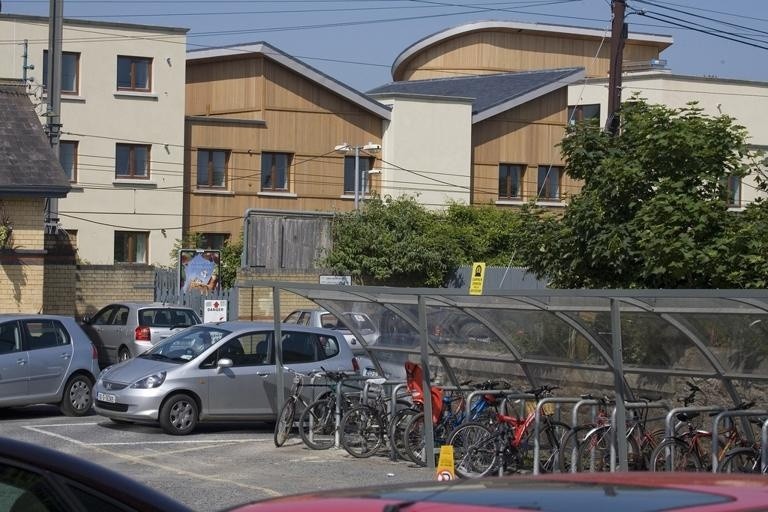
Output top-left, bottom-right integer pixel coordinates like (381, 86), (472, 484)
(526, 397), (555, 415)
(493, 381), (511, 397)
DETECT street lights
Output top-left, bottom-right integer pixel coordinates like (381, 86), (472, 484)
(361, 169), (382, 206)
(334, 142), (383, 216)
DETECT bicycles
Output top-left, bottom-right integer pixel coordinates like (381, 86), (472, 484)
(718, 417), (765, 475)
(273, 364), (668, 472)
(650, 400), (758, 473)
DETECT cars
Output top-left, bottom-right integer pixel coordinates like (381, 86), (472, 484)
(283, 310), (381, 356)
(222, 471), (768, 511)
(90, 322), (362, 434)
(0, 311), (103, 417)
(1, 436), (197, 512)
(83, 290), (205, 369)
